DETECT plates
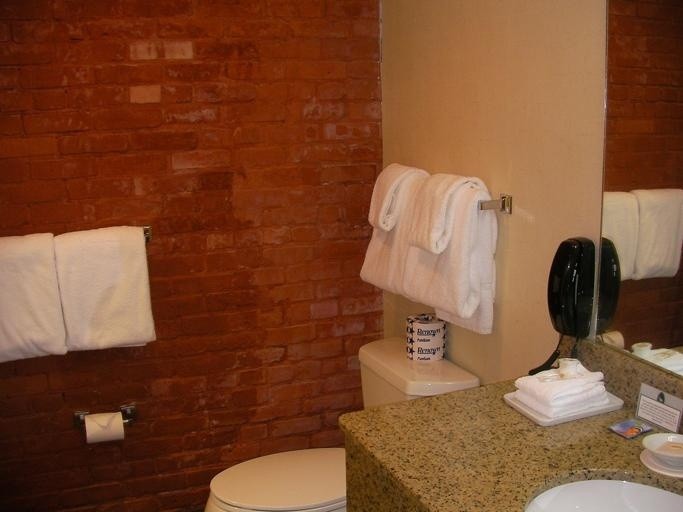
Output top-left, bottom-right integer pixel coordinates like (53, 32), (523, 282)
(640, 449), (683, 479)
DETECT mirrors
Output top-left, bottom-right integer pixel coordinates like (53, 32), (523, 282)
(593, 1), (682, 381)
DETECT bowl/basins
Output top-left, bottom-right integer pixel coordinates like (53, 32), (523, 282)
(642, 433), (683, 467)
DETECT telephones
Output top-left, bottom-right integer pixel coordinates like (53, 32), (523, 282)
(596, 237), (620, 335)
(547, 236), (595, 338)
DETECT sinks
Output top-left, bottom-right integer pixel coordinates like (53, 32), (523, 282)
(527, 469), (682, 511)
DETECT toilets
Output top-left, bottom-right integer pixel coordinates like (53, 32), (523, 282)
(204, 334), (479, 510)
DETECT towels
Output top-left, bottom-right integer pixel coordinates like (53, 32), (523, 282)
(597, 187), (682, 284)
(513, 359), (607, 419)
(357, 161), (499, 337)
(1, 224), (158, 362)
